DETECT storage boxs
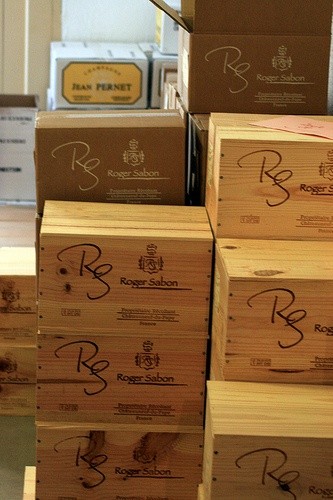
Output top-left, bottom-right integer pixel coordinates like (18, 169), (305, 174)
(0, 0), (333, 500)
